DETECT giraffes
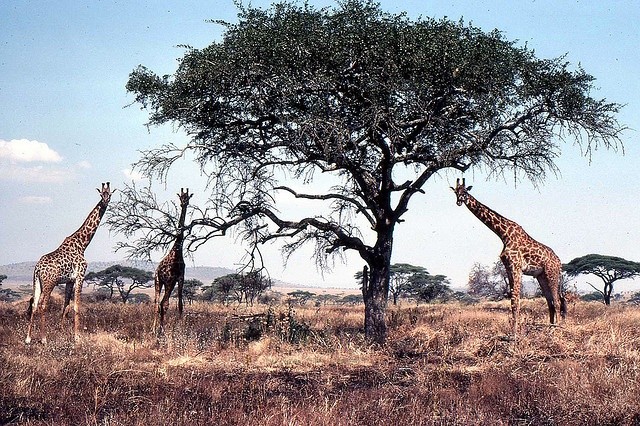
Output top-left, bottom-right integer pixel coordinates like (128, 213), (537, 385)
(150, 188), (193, 335)
(24, 182), (116, 345)
(450, 178), (566, 332)
